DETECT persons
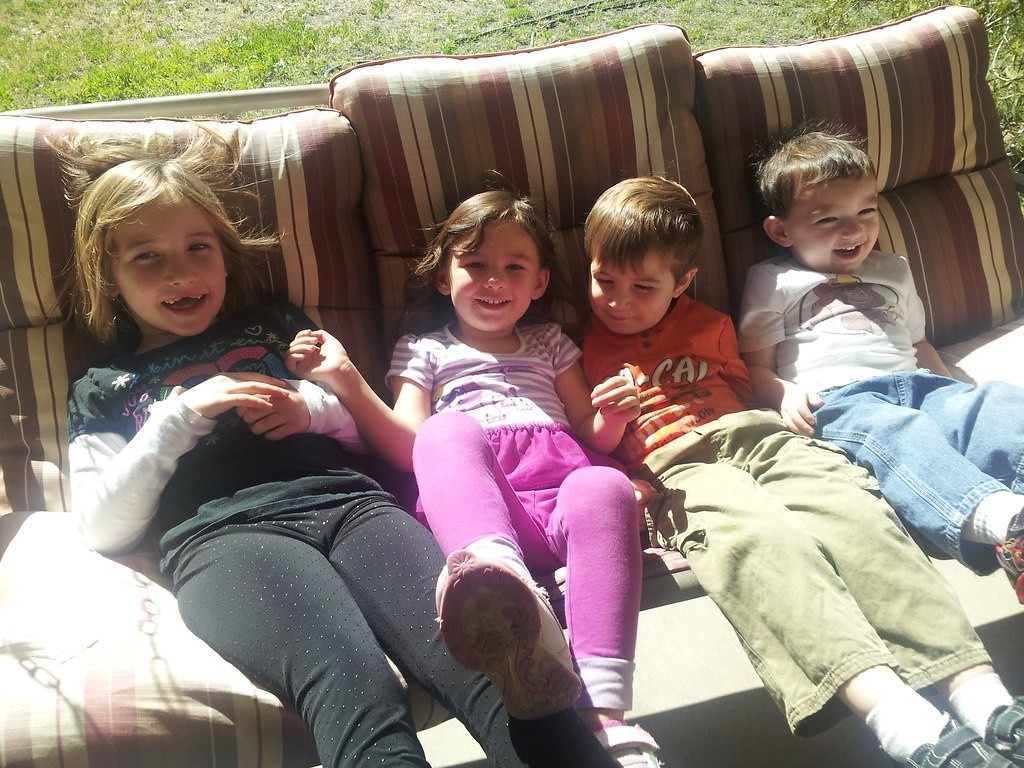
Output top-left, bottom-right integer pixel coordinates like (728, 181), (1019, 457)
(284, 167), (665, 768)
(734, 115), (1024, 606)
(43, 120), (626, 768)
(578, 175), (1024, 768)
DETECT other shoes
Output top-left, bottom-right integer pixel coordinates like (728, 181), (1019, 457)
(982, 693), (1024, 768)
(994, 506), (1024, 604)
(904, 709), (1020, 768)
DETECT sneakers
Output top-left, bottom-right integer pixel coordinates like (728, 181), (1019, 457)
(436, 550), (582, 721)
(593, 723), (661, 768)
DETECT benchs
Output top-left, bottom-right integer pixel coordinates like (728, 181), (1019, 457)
(1, 5), (1022, 767)
